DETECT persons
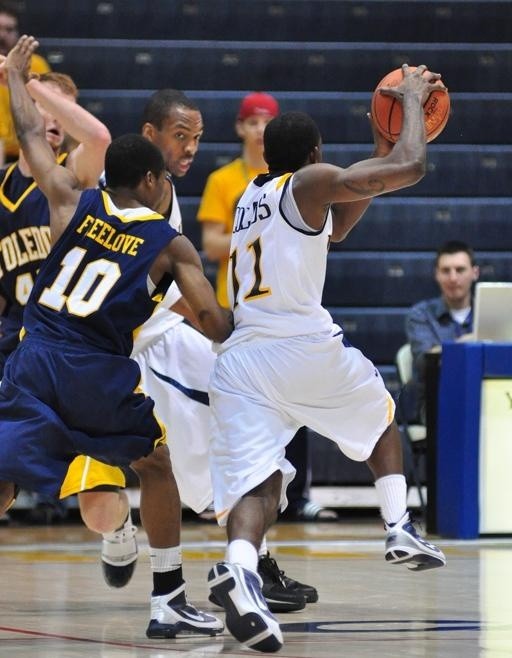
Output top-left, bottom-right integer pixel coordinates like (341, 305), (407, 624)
(103, 84), (320, 612)
(1, 72), (114, 531)
(0, 1), (21, 58)
(406, 238), (481, 427)
(285, 426), (341, 521)
(203, 62), (448, 654)
(195, 89), (282, 318)
(1, 31), (232, 643)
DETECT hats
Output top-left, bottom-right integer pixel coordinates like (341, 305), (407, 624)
(240, 92), (280, 122)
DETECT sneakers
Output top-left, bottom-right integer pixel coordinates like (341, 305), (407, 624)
(381, 513), (445, 572)
(147, 551), (318, 652)
(101, 507), (139, 587)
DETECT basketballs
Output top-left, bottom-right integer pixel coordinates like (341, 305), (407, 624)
(371, 68), (451, 144)
